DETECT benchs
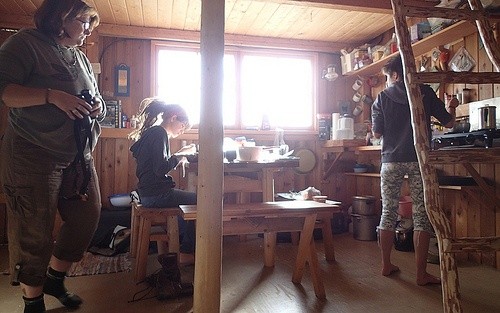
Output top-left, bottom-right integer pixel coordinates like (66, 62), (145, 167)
(130, 193), (339, 301)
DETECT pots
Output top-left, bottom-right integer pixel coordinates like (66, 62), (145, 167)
(395, 218), (415, 252)
(349, 213), (377, 241)
(351, 195), (376, 215)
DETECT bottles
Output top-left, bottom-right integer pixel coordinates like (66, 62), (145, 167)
(121, 115), (128, 128)
(341, 114), (354, 140)
(445, 94), (462, 109)
(462, 88), (469, 104)
(130, 115), (136, 129)
(247, 139), (255, 146)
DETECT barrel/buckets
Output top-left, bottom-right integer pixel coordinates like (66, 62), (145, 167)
(398, 195), (412, 218)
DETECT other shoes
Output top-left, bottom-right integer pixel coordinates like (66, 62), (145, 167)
(157, 283), (193, 299)
(43, 277), (82, 307)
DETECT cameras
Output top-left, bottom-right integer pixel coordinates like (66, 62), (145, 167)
(79, 89), (92, 105)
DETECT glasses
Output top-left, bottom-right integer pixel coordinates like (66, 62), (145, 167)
(76, 18), (91, 31)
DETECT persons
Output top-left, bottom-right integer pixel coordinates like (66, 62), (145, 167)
(371, 56), (460, 286)
(128, 99), (197, 263)
(0, 0), (107, 313)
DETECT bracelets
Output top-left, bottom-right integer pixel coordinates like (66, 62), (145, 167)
(449, 106), (455, 109)
(46, 88), (51, 104)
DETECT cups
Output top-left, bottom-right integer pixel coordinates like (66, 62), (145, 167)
(478, 104), (497, 129)
(352, 79), (362, 91)
(226, 151), (237, 162)
(313, 196), (327, 203)
(352, 92), (362, 102)
(353, 104), (362, 116)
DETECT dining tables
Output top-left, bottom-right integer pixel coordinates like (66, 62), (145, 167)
(179, 156), (300, 268)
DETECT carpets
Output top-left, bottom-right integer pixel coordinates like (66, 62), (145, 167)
(68, 254), (131, 277)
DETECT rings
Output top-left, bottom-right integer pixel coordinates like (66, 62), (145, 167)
(72, 110), (76, 113)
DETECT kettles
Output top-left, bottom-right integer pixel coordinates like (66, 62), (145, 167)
(455, 115), (470, 132)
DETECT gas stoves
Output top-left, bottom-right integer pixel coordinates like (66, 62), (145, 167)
(434, 128), (500, 148)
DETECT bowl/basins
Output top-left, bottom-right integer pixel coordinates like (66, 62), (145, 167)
(107, 194), (132, 207)
(291, 146), (318, 176)
(353, 167), (369, 173)
(239, 147), (261, 161)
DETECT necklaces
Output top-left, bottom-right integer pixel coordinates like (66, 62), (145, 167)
(57, 45), (76, 66)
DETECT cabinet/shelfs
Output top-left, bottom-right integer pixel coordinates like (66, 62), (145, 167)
(325, 18), (499, 208)
